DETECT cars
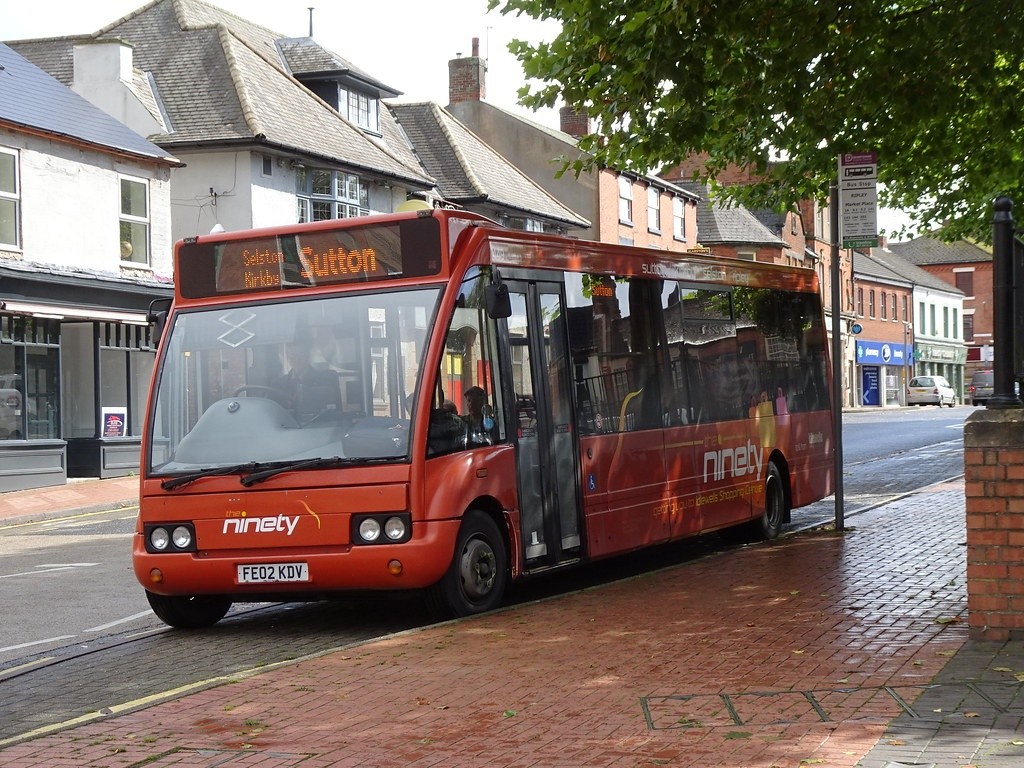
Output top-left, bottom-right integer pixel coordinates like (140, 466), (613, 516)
(906, 375), (956, 409)
(969, 369), (996, 405)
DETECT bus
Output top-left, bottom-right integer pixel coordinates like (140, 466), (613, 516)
(130, 206), (840, 627)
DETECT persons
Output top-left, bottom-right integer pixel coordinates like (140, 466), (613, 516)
(261, 343), (334, 421)
(403, 387), (500, 447)
